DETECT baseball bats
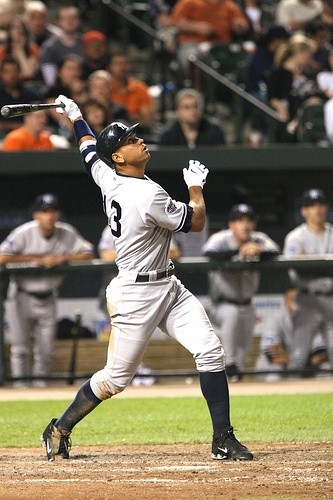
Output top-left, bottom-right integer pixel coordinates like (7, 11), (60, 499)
(1, 102), (66, 119)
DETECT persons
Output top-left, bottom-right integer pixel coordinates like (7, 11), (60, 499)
(42, 95), (255, 461)
(0, 0), (333, 391)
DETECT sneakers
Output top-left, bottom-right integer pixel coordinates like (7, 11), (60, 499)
(39, 418), (73, 461)
(210, 425), (253, 460)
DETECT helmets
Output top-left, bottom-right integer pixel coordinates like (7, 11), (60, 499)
(33, 194), (60, 212)
(94, 120), (140, 168)
(227, 203), (254, 220)
(299, 190), (327, 208)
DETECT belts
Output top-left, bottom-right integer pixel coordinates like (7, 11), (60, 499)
(135, 261), (175, 282)
(216, 296), (250, 305)
(300, 287), (332, 296)
(19, 289), (54, 298)
